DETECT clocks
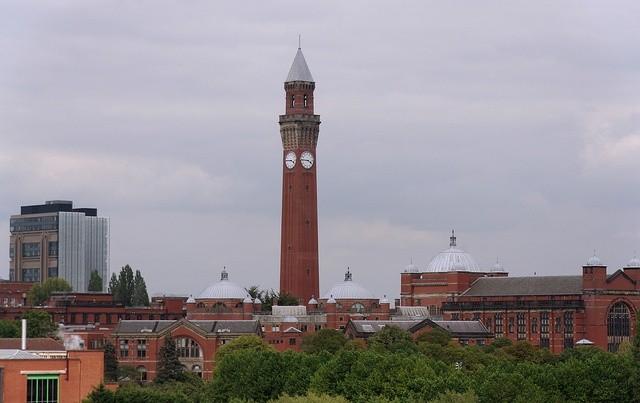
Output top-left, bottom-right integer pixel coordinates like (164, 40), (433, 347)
(285, 150), (297, 170)
(299, 151), (314, 169)
